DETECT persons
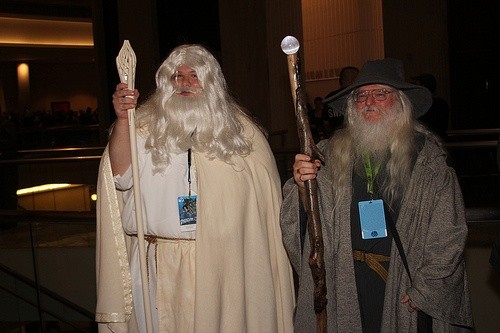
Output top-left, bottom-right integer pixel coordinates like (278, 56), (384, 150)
(305, 66), (449, 166)
(0, 107), (99, 160)
(279, 58), (475, 333)
(94, 45), (296, 333)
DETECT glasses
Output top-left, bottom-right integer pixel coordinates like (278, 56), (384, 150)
(352, 87), (395, 103)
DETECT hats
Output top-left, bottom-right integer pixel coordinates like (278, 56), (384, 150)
(321, 59), (432, 119)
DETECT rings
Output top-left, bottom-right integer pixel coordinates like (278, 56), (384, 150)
(299, 174), (303, 182)
(297, 168), (301, 174)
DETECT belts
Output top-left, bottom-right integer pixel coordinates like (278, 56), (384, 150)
(354, 250), (392, 283)
